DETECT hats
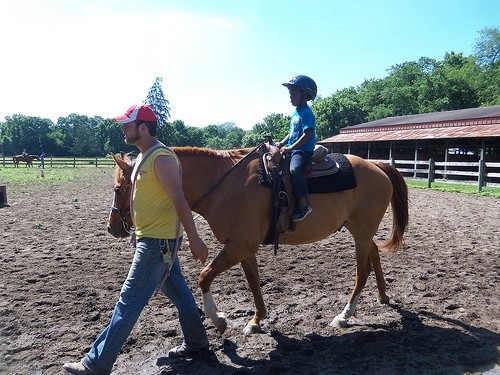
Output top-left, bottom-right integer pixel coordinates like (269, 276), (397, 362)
(115, 105), (159, 123)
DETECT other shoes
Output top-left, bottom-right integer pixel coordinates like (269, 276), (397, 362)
(291, 205), (312, 221)
(168, 345), (210, 357)
(63, 362), (92, 375)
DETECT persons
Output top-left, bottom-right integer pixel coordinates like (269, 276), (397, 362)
(275, 75), (318, 222)
(22, 149), (29, 158)
(38, 152), (46, 169)
(61, 105), (210, 375)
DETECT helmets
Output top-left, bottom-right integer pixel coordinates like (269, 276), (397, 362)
(282, 75), (317, 101)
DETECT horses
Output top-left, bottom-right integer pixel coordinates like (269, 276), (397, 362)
(106, 146), (410, 334)
(12, 155), (36, 168)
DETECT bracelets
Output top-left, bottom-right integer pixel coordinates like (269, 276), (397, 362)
(188, 236), (199, 239)
